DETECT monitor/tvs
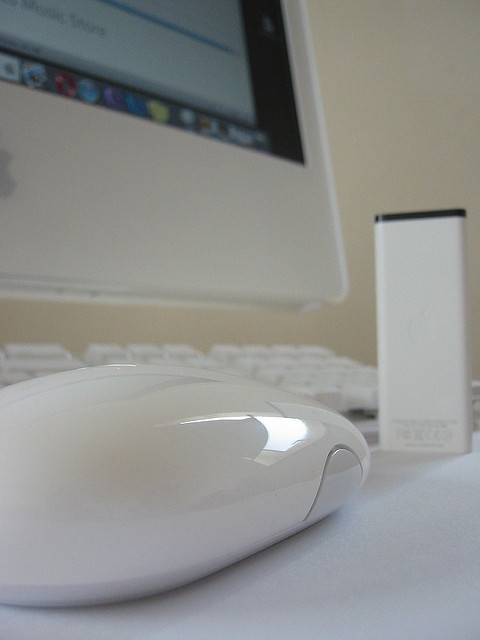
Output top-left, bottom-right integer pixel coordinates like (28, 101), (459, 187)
(0, 1), (349, 308)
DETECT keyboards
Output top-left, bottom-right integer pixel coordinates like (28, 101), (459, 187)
(0, 342), (379, 412)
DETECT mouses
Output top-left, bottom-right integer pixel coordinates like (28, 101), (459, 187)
(0, 359), (371, 608)
